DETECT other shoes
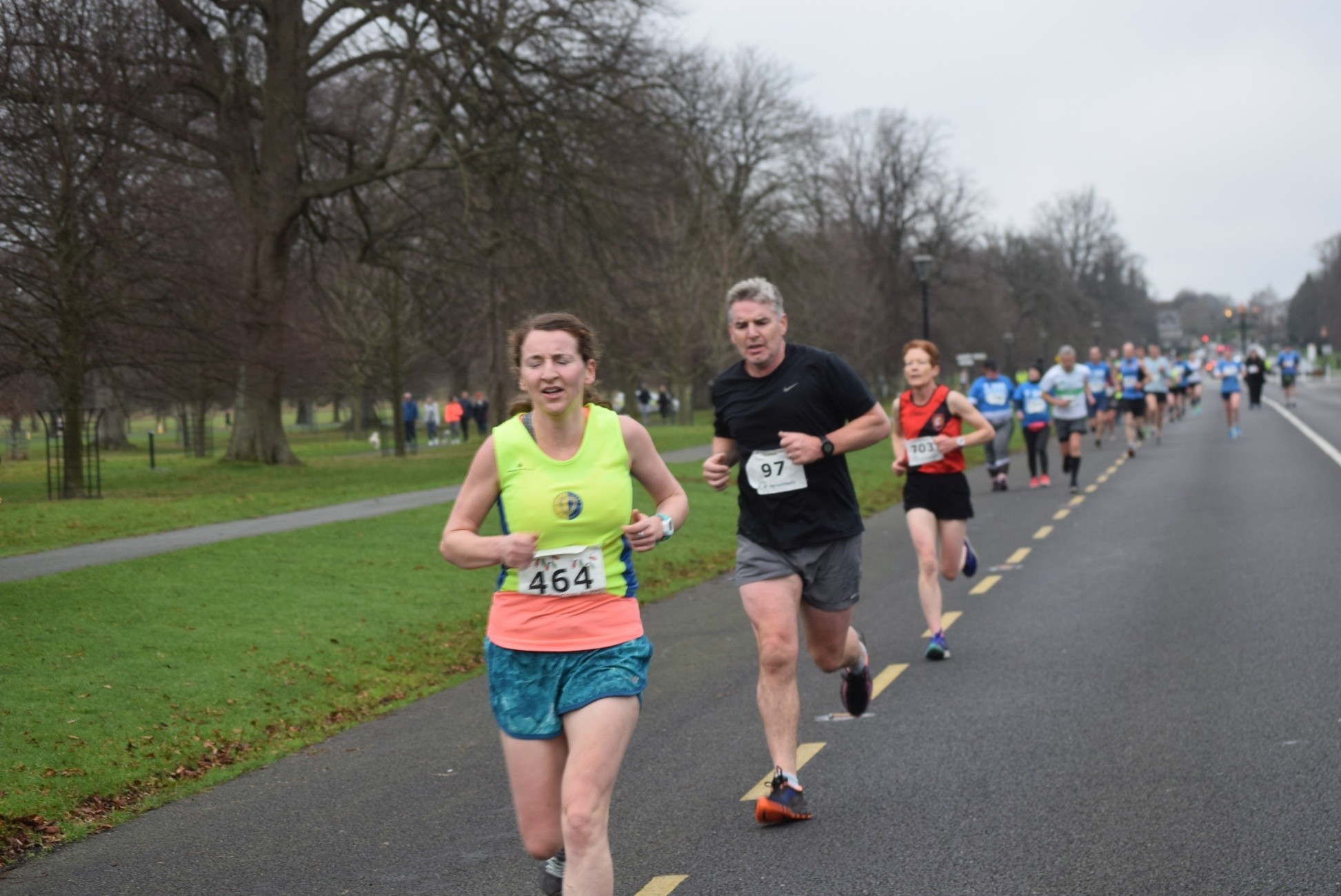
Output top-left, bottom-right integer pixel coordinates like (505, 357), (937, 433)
(1064, 461), (1070, 474)
(1157, 436), (1162, 444)
(1030, 476), (1038, 488)
(1228, 427), (1240, 438)
(1149, 426), (1155, 436)
(1137, 425), (1146, 440)
(1109, 433), (1116, 441)
(1040, 474), (1050, 487)
(1091, 426), (1096, 433)
(992, 477), (999, 492)
(1095, 439), (1101, 449)
(1070, 483), (1079, 493)
(998, 472), (1009, 492)
(1129, 445), (1136, 457)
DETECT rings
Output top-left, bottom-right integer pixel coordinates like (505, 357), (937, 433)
(639, 532), (645, 538)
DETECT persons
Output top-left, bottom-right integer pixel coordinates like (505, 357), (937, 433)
(890, 340), (996, 660)
(1212, 349), (1246, 439)
(438, 311), (690, 896)
(638, 383), (676, 426)
(1107, 341), (1202, 458)
(1084, 346), (1122, 445)
(444, 390), (489, 444)
(959, 368), (969, 392)
(1243, 351), (1266, 409)
(702, 276), (892, 820)
(424, 397), (441, 446)
(1276, 346), (1300, 405)
(404, 393), (419, 454)
(1039, 344), (1096, 493)
(1013, 358), (1056, 489)
(968, 359), (1016, 491)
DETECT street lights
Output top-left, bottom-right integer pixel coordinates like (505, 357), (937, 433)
(910, 252), (942, 339)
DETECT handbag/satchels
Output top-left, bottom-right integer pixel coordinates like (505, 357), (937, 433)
(1028, 421), (1045, 432)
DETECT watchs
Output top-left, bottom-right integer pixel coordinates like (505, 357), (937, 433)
(819, 436), (835, 458)
(956, 436), (965, 448)
(656, 512), (674, 544)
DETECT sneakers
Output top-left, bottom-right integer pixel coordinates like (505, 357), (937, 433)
(755, 766), (813, 825)
(541, 847), (565, 895)
(924, 637), (952, 661)
(840, 629), (873, 717)
(963, 538), (978, 577)
